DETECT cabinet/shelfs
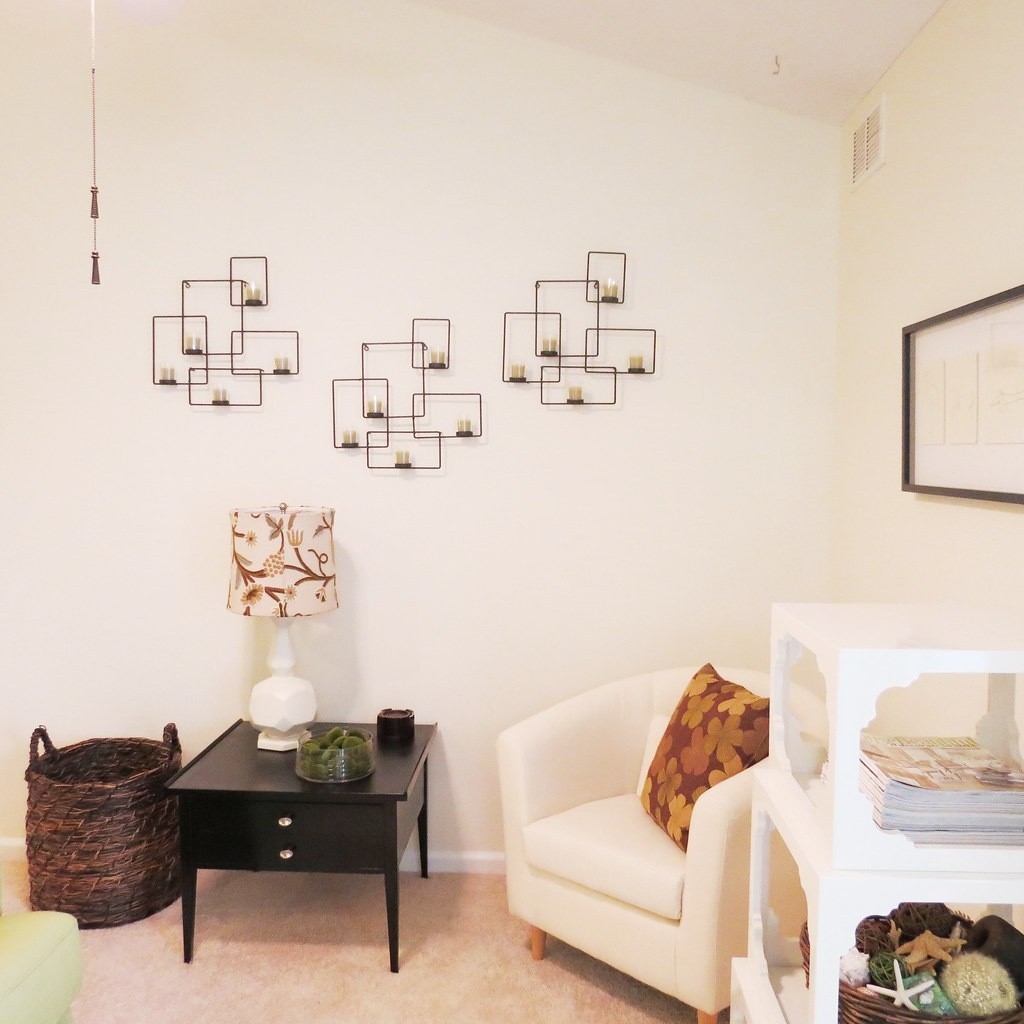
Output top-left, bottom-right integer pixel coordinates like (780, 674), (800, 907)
(730, 600), (1024, 1024)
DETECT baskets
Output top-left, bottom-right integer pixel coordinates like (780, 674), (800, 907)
(799, 919), (1024, 1024)
(25, 724), (185, 928)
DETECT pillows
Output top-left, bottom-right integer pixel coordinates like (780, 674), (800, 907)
(637, 663), (770, 852)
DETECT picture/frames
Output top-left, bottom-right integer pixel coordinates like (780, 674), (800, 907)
(902, 284), (1024, 503)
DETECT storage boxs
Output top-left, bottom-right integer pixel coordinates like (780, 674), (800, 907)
(24, 723), (187, 929)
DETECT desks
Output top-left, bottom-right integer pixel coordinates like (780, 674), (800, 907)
(162, 719), (438, 973)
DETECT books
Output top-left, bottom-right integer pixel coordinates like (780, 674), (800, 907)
(853, 736), (1024, 849)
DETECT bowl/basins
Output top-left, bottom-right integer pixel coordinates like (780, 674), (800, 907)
(296, 726), (374, 782)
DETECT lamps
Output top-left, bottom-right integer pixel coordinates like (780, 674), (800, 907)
(225, 503), (340, 752)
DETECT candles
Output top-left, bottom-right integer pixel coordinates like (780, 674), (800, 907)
(511, 364), (518, 378)
(213, 388), (221, 400)
(637, 356), (643, 367)
(351, 432), (357, 443)
(187, 336), (193, 348)
(457, 417), (464, 431)
(611, 279), (618, 298)
(551, 338), (556, 351)
(343, 432), (350, 443)
(630, 355), (636, 368)
(194, 336), (200, 348)
(275, 357), (281, 369)
(282, 357), (289, 368)
(169, 368), (174, 380)
(404, 450), (410, 463)
(396, 451), (403, 463)
(376, 401), (382, 413)
(431, 351), (437, 362)
(576, 387), (582, 399)
(438, 352), (444, 363)
(464, 417), (471, 431)
(162, 368), (167, 380)
(254, 288), (261, 300)
(221, 388), (228, 401)
(603, 279), (610, 297)
(368, 400), (374, 413)
(543, 339), (549, 351)
(569, 387), (574, 399)
(247, 288), (253, 299)
(519, 364), (525, 378)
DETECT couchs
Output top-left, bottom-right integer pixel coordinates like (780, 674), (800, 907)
(1, 910), (85, 1024)
(496, 662), (808, 1024)
